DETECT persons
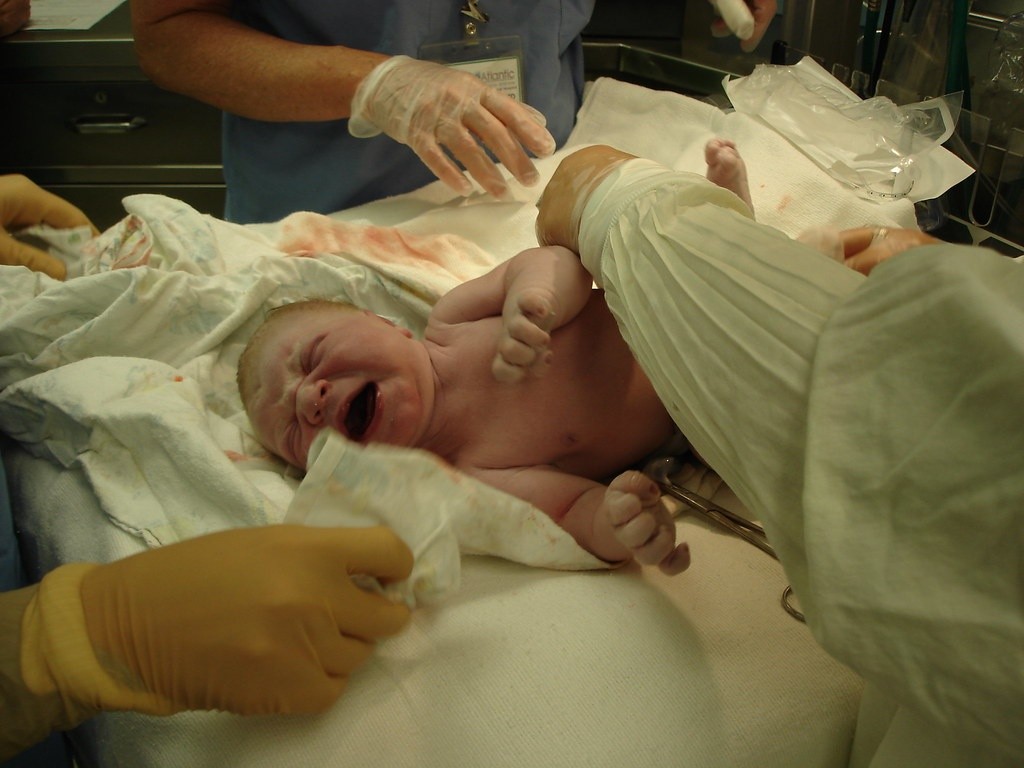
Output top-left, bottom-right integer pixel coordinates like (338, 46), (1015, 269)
(234, 138), (756, 576)
(127, 0), (778, 227)
(0, 171), (416, 756)
(537, 143), (1024, 768)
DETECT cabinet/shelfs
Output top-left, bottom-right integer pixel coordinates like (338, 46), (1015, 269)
(1, 81), (227, 235)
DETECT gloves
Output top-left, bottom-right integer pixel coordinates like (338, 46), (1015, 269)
(1, 170), (104, 282)
(16, 525), (416, 719)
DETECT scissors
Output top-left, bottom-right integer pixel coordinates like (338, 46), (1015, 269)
(658, 480), (807, 621)
